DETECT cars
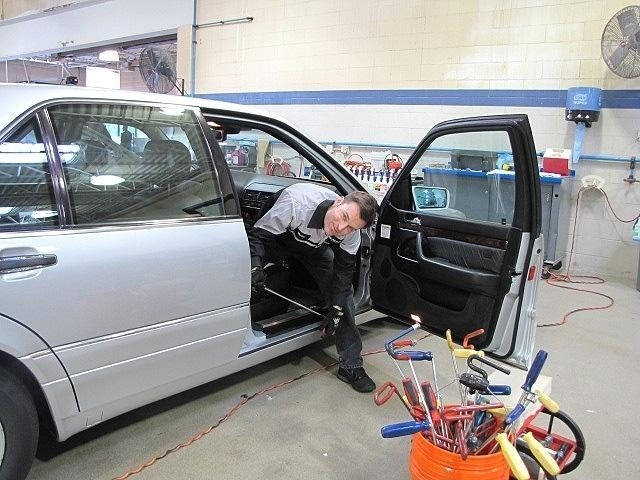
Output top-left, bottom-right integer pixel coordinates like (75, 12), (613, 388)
(0, 82), (545, 479)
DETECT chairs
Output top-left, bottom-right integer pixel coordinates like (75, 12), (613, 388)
(83, 141), (109, 165)
(142, 140), (291, 318)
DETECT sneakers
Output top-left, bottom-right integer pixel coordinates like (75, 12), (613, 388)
(337, 366), (376, 393)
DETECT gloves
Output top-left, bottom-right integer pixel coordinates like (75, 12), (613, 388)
(251, 266), (268, 292)
(317, 304), (344, 338)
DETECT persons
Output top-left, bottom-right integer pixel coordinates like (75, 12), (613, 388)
(118, 130), (133, 152)
(247, 181), (377, 393)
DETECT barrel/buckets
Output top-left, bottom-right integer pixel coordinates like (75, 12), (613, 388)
(409, 404), (515, 480)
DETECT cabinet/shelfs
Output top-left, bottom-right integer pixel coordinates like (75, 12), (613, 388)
(423, 165), (576, 277)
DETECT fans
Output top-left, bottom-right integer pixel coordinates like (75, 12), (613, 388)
(602, 5), (640, 78)
(138, 45), (185, 96)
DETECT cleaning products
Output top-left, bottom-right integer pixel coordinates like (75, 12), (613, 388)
(346, 164), (401, 196)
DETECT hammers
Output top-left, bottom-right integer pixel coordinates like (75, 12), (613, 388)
(264, 284), (340, 336)
